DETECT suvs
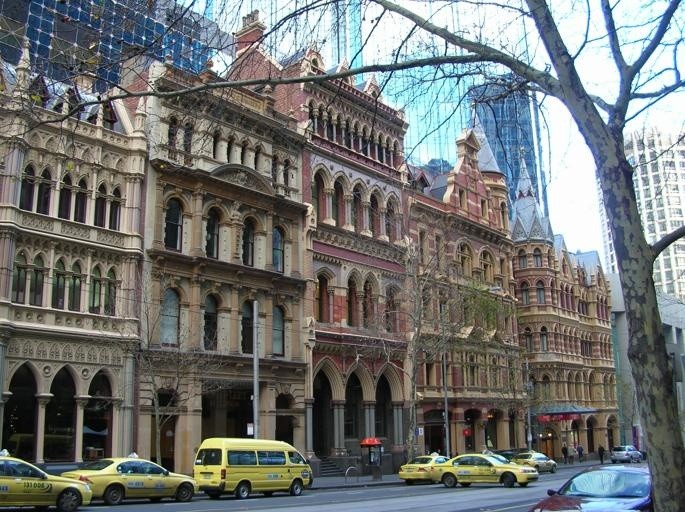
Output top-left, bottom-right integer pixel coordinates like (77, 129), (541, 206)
(610, 445), (644, 463)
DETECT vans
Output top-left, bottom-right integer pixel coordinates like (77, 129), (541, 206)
(192, 437), (315, 498)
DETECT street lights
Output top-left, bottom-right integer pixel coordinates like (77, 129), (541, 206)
(539, 432), (554, 457)
(441, 285), (501, 458)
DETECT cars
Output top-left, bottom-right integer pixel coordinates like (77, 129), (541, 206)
(431, 444), (540, 488)
(528, 464), (654, 511)
(509, 449), (558, 474)
(0, 448), (93, 511)
(398, 450), (453, 485)
(60, 452), (198, 506)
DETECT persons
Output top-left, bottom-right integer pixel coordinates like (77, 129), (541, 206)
(598, 444), (606, 465)
(568, 444), (577, 465)
(561, 444), (569, 464)
(576, 443), (585, 465)
